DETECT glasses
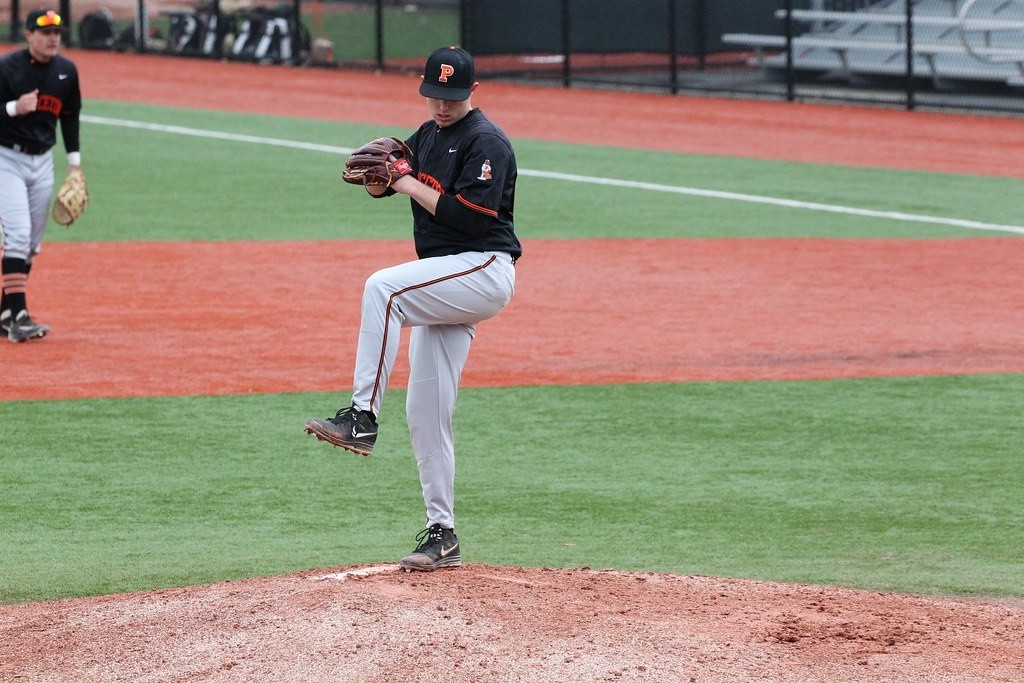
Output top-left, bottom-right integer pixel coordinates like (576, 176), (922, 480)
(29, 14), (64, 29)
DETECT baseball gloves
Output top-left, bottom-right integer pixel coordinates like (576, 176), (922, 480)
(340, 135), (417, 199)
(51, 171), (89, 226)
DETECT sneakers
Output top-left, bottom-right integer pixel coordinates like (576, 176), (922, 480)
(400, 524), (462, 571)
(304, 402), (378, 456)
(8, 309), (49, 343)
(0, 308), (13, 336)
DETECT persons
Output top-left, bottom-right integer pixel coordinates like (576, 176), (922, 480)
(0, 8), (88, 342)
(305, 46), (523, 572)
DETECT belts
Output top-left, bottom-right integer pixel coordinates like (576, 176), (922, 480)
(0, 139), (51, 155)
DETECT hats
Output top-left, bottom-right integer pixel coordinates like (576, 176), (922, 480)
(26, 9), (64, 32)
(419, 45), (473, 101)
